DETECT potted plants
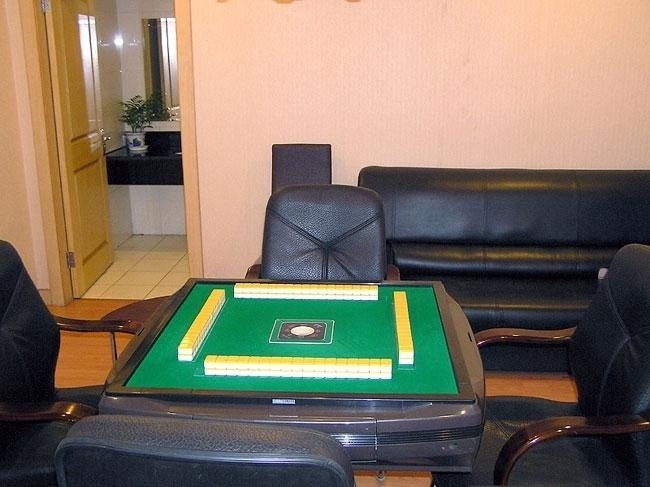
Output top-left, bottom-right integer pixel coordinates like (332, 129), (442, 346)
(118, 93), (174, 150)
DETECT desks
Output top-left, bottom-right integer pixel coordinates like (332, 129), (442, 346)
(99, 277), (487, 474)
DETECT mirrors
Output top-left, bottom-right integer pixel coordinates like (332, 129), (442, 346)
(140, 17), (182, 122)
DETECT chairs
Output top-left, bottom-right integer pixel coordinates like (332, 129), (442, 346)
(429, 238), (648, 487)
(259, 183), (388, 283)
(2, 238), (145, 487)
(52, 412), (356, 487)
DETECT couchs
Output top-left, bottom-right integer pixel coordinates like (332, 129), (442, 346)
(358, 165), (648, 370)
